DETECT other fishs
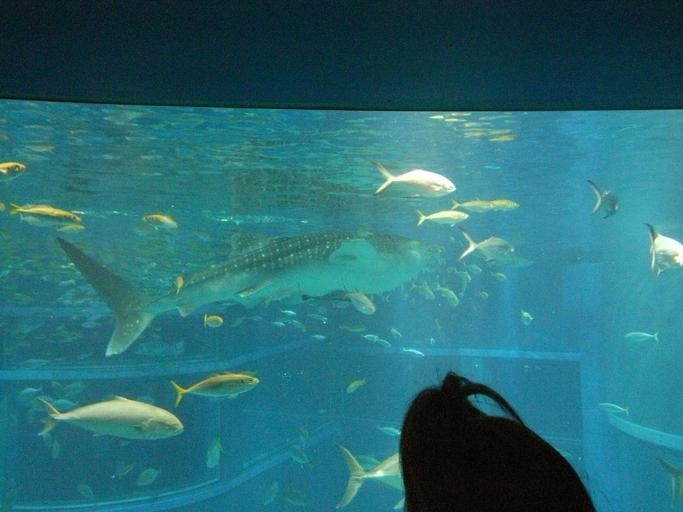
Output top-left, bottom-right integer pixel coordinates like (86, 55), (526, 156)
(0, 100), (520, 512)
